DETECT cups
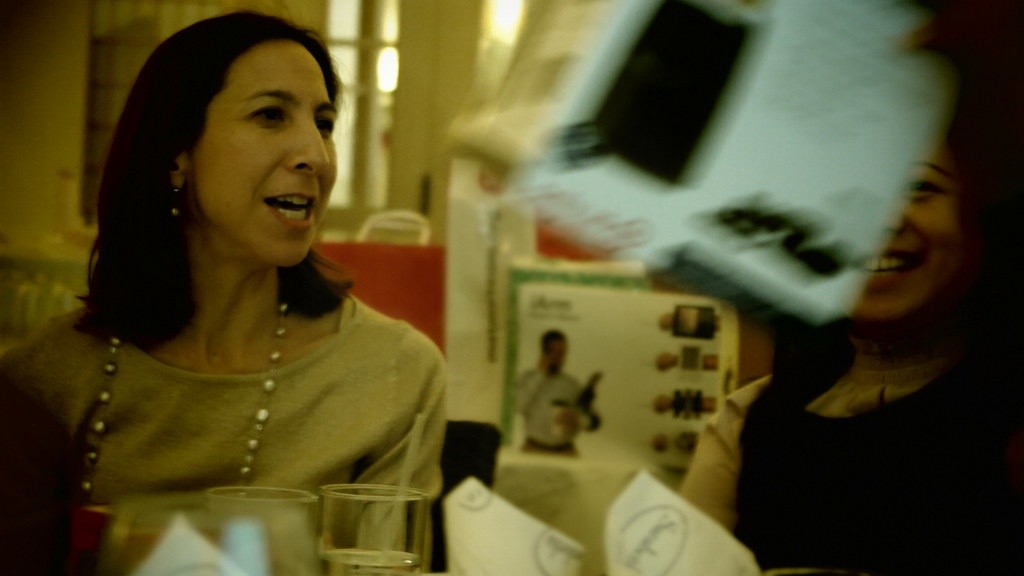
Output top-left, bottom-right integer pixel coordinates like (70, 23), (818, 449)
(203, 486), (317, 576)
(315, 484), (433, 576)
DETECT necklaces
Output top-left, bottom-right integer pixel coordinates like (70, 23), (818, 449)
(79, 301), (288, 506)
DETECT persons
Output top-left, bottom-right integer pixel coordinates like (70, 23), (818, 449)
(514, 330), (602, 453)
(0, 6), (449, 576)
(678, 100), (1024, 576)
